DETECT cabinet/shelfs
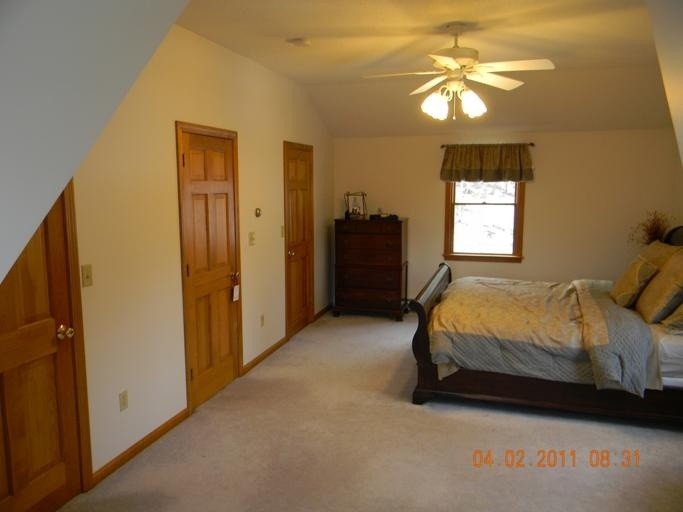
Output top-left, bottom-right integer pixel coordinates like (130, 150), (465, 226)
(332, 218), (408, 321)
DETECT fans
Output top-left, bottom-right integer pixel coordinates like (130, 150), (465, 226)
(361, 23), (554, 121)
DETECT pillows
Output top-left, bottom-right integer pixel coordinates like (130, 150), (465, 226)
(609, 240), (681, 326)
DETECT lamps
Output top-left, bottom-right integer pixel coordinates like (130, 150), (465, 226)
(418, 79), (487, 121)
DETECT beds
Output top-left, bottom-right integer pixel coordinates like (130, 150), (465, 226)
(408, 226), (681, 433)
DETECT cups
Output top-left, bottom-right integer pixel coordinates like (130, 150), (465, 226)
(378, 208), (390, 218)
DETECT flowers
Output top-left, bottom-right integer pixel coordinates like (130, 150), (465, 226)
(628, 210), (678, 244)
